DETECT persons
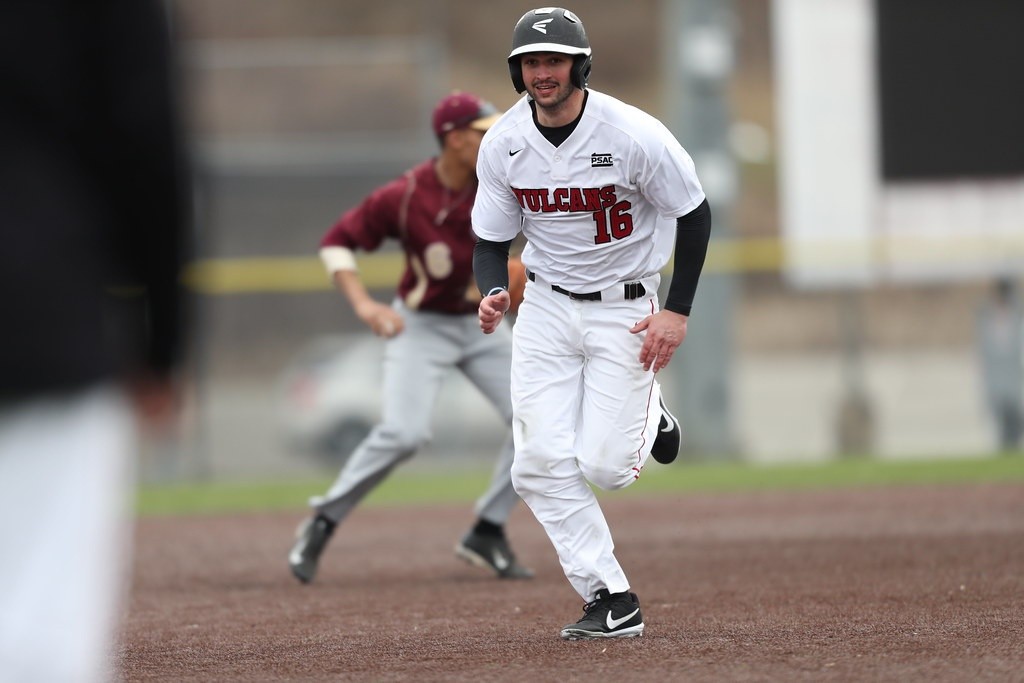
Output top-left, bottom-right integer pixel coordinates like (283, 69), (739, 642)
(470, 7), (713, 641)
(286, 91), (539, 587)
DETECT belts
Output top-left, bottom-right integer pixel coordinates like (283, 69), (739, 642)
(525, 270), (645, 301)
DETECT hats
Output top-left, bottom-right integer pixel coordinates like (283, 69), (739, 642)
(430, 93), (505, 136)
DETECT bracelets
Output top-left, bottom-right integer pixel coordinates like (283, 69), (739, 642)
(486, 285), (507, 298)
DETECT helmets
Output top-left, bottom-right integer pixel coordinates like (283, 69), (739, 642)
(508, 7), (592, 95)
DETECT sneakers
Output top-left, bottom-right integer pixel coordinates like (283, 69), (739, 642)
(650, 391), (681, 466)
(289, 510), (334, 582)
(560, 590), (644, 640)
(453, 527), (535, 581)
